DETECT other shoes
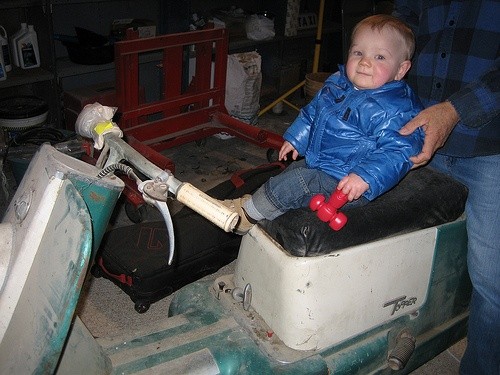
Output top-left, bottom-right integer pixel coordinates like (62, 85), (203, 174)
(216, 194), (256, 236)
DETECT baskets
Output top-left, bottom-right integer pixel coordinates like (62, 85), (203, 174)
(305, 72), (333, 98)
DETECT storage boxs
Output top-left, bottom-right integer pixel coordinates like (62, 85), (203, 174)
(65, 88), (118, 132)
(56, 55), (116, 93)
(232, 213), (438, 349)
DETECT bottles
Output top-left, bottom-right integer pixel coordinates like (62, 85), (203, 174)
(0, 22), (41, 81)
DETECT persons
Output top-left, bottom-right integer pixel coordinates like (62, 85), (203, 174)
(217, 14), (426, 237)
(392, 0), (500, 375)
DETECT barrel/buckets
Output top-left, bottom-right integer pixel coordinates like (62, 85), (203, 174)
(0, 96), (49, 145)
(303, 72), (330, 102)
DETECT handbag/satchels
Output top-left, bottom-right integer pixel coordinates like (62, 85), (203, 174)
(246, 14), (275, 40)
(224, 52), (262, 127)
